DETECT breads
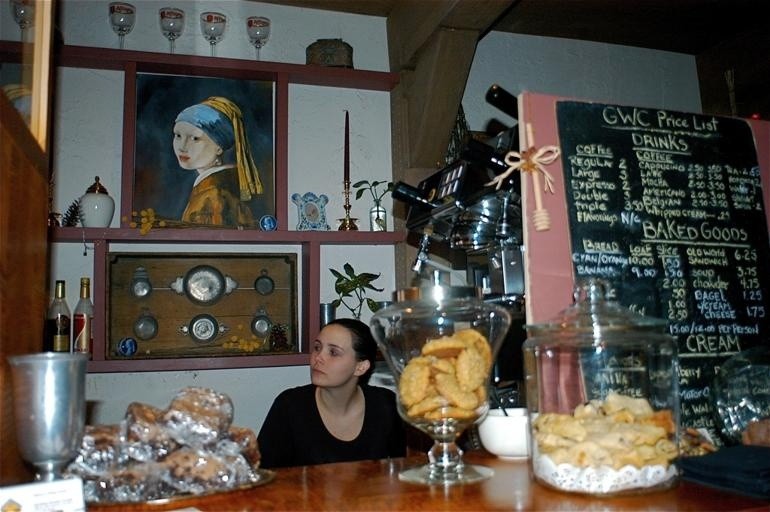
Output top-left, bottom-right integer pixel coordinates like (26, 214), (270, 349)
(535, 390), (681, 469)
(64, 387), (260, 506)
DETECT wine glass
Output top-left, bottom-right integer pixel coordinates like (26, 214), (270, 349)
(370, 296), (513, 487)
(7, 350), (90, 482)
(159, 7), (184, 55)
(108, 1), (137, 50)
(200, 12), (226, 57)
(10, 0), (35, 43)
(246, 16), (272, 61)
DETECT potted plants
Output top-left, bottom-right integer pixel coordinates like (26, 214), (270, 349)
(351, 178), (396, 231)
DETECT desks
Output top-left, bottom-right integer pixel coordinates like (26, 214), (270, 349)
(84, 446), (770, 512)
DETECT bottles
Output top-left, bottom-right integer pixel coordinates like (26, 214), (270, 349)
(370, 198), (386, 232)
(517, 270), (686, 503)
(44, 279), (70, 354)
(73, 276), (94, 362)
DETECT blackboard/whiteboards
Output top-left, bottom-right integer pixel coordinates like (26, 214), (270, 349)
(555, 101), (770, 450)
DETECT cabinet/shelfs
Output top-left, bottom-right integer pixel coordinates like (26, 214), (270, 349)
(50, 44), (407, 374)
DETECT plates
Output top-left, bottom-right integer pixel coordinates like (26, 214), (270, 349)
(85, 467), (277, 512)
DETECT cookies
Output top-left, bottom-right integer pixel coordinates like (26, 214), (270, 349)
(399, 328), (493, 422)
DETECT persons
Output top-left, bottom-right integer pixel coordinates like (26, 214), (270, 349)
(257, 318), (435, 468)
(173, 96), (264, 225)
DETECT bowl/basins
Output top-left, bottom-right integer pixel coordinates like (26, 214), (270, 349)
(478, 407), (533, 460)
